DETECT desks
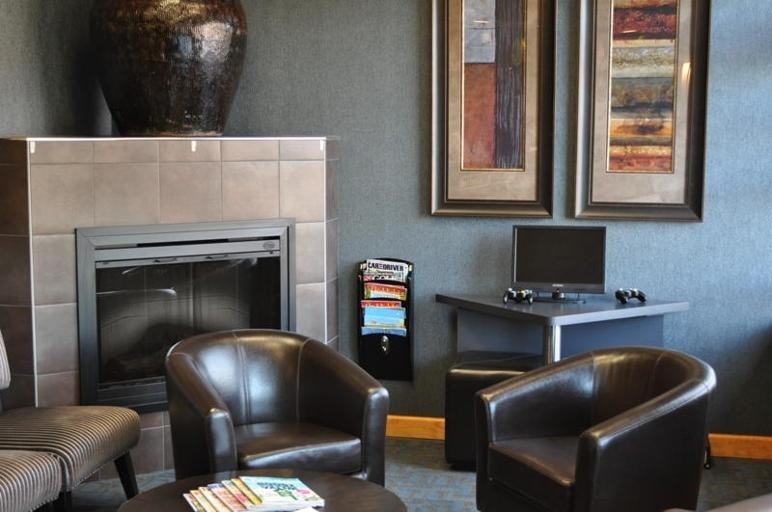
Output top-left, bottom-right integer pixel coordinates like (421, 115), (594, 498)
(430, 291), (692, 369)
(116, 466), (408, 512)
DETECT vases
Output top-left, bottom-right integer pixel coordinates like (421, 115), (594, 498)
(81, 0), (249, 140)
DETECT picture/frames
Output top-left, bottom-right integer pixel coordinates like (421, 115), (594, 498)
(426, 0), (559, 221)
(567, 0), (715, 227)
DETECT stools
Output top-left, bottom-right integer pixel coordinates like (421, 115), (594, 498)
(444, 346), (549, 474)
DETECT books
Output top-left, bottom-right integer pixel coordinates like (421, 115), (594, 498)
(358, 258), (409, 337)
(182, 474), (325, 512)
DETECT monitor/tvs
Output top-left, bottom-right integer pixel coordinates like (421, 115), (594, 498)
(513, 224), (605, 303)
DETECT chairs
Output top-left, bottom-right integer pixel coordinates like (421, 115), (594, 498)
(0, 328), (143, 512)
(469, 345), (719, 511)
(163, 328), (391, 491)
(1, 451), (62, 512)
